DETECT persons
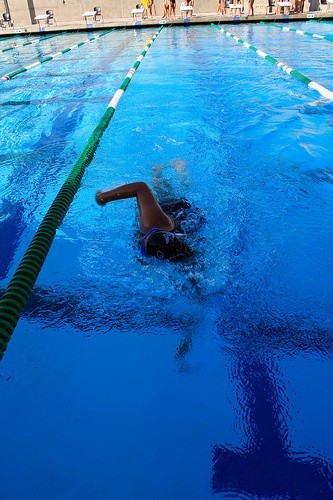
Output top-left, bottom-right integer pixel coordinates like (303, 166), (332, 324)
(217, 0), (254, 16)
(162, 0), (176, 19)
(184, 0), (194, 19)
(139, 0), (156, 18)
(96, 181), (192, 264)
(275, 0), (304, 15)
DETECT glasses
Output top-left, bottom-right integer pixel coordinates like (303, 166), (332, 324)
(141, 228), (161, 256)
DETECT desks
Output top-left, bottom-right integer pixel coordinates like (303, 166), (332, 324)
(83, 11), (97, 22)
(277, 1), (291, 14)
(33, 15), (49, 26)
(180, 6), (193, 19)
(229, 4), (244, 17)
(131, 9), (144, 20)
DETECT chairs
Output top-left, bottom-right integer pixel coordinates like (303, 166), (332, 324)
(2, 13), (13, 27)
(45, 10), (57, 26)
(93, 7), (103, 21)
(222, 0), (233, 15)
(266, 0), (276, 15)
(136, 4), (145, 13)
